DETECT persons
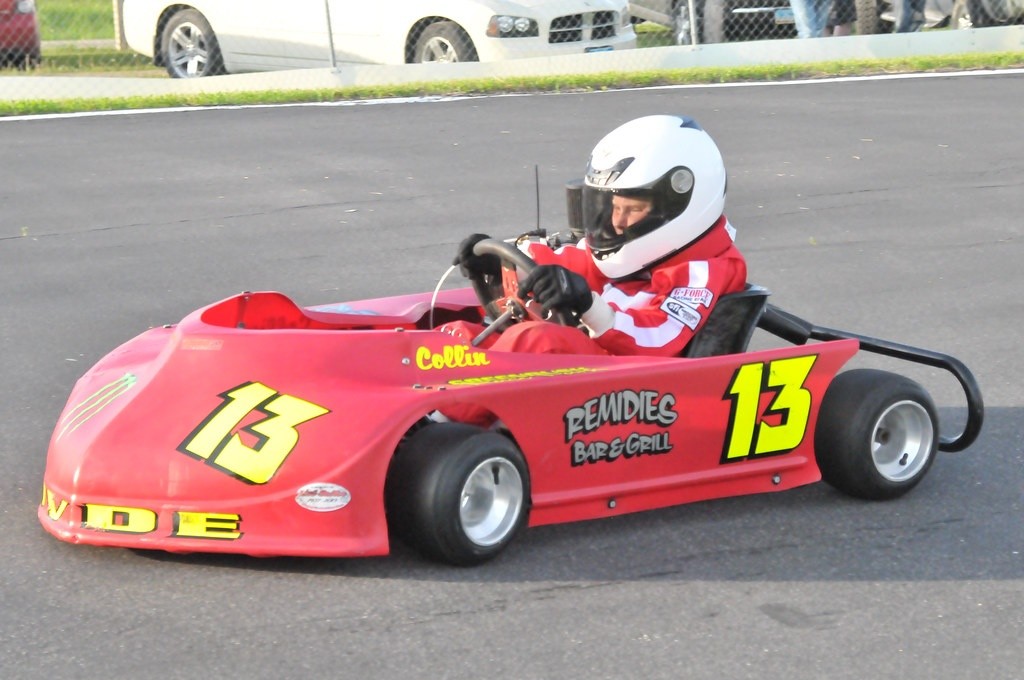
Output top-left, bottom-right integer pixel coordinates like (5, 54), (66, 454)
(791, 0), (856, 39)
(435, 116), (747, 356)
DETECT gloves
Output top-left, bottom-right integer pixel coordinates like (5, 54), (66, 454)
(518, 264), (592, 319)
(452, 234), (500, 280)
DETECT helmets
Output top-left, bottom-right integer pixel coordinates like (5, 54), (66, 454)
(581, 114), (729, 279)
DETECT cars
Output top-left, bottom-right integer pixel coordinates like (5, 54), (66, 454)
(0, 0), (39, 66)
(121, 0), (638, 79)
